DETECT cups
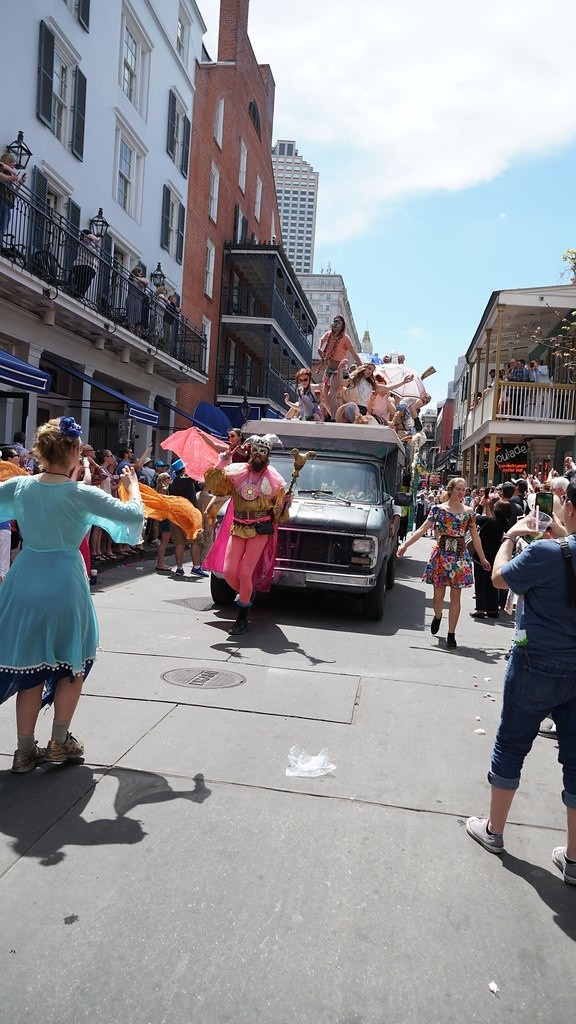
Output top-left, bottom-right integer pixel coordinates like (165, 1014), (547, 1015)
(529, 510), (553, 540)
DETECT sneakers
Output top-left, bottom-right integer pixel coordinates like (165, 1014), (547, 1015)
(466, 816), (504, 853)
(11, 739), (48, 772)
(176, 568), (184, 576)
(45, 729), (85, 763)
(551, 846), (576, 884)
(190, 566), (209, 577)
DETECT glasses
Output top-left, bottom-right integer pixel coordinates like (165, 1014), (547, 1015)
(560, 493), (569, 504)
(367, 366), (374, 372)
(10, 453), (20, 459)
(228, 434), (238, 439)
(251, 445), (269, 455)
(299, 378), (309, 383)
(87, 449), (133, 458)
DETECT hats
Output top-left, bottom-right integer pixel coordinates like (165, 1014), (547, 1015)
(502, 481), (515, 497)
(511, 478), (528, 492)
(143, 457), (186, 474)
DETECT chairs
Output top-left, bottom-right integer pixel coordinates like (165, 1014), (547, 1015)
(2, 233), (27, 268)
(33, 250), (73, 295)
(97, 293), (126, 326)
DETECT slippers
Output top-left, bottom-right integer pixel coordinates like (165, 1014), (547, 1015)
(90, 538), (162, 561)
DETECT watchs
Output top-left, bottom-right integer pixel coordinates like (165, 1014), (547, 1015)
(501, 532), (516, 544)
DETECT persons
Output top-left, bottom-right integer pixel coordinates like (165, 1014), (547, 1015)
(0, 154), (27, 244)
(467, 483), (576, 884)
(126, 268), (180, 352)
(0, 417), (146, 773)
(469, 359), (551, 421)
(396, 478), (491, 649)
(284, 354), (432, 445)
(359, 471), (401, 537)
(318, 315), (363, 422)
(205, 434), (294, 634)
(461, 457), (576, 660)
(73, 229), (100, 300)
(415, 488), (447, 542)
(160, 427), (248, 484)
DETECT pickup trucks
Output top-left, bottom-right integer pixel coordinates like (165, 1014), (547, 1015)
(210, 418), (413, 622)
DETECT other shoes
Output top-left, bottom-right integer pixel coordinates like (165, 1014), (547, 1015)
(538, 721), (557, 740)
(431, 613), (443, 635)
(484, 611), (498, 618)
(155, 564), (172, 572)
(447, 632), (456, 649)
(468, 611), (484, 619)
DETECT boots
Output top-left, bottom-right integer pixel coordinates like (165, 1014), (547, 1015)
(249, 588), (271, 621)
(229, 599), (252, 634)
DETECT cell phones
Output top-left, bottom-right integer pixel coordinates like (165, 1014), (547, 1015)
(536, 493), (553, 530)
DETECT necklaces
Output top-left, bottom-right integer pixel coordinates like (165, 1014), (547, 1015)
(316, 333), (344, 374)
(42, 469), (70, 478)
(0, 432), (218, 579)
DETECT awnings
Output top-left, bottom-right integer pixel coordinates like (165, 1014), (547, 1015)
(193, 402), (233, 433)
(156, 398), (228, 441)
(41, 356), (160, 428)
(0, 349), (52, 395)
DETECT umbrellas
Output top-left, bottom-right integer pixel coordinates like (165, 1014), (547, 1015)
(375, 364), (426, 399)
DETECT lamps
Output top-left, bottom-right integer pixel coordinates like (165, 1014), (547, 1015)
(150, 262), (166, 286)
(120, 348), (130, 364)
(89, 207), (110, 237)
(94, 335), (105, 350)
(6, 131), (33, 169)
(43, 310), (55, 326)
(145, 360), (154, 374)
